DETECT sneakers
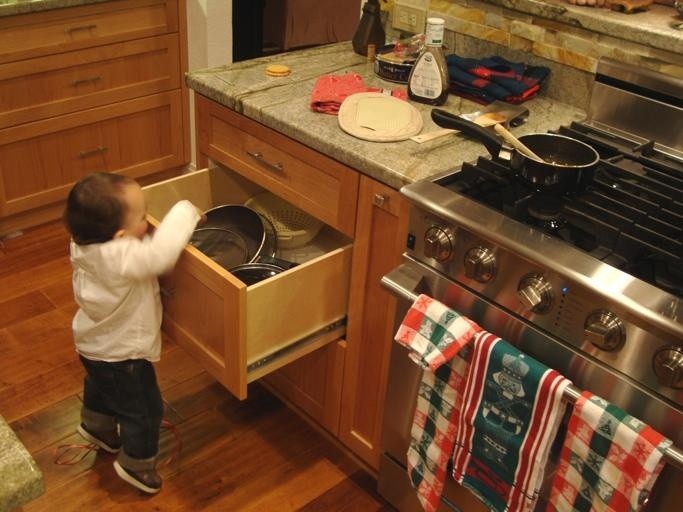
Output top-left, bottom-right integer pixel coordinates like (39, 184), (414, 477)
(77, 420), (122, 453)
(112, 458), (163, 494)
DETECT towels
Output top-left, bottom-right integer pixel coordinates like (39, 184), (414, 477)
(451, 332), (573, 511)
(545, 389), (673, 512)
(393, 293), (484, 508)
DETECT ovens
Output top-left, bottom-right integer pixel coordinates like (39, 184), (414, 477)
(376, 189), (683, 512)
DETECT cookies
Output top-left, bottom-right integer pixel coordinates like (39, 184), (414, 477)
(265, 65), (290, 77)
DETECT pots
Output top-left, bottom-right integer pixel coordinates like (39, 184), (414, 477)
(428, 104), (601, 198)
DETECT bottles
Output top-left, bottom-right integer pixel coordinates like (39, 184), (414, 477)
(407, 17), (451, 108)
(351, 0), (387, 55)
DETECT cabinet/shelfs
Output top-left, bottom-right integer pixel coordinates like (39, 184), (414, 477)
(124, 94), (361, 452)
(1, 0), (194, 237)
(337, 178), (407, 476)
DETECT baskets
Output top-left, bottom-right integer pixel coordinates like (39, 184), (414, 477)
(243, 193), (325, 250)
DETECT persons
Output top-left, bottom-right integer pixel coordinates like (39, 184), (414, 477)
(63, 173), (208, 492)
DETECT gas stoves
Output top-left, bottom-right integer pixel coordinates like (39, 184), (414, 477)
(431, 119), (683, 299)
